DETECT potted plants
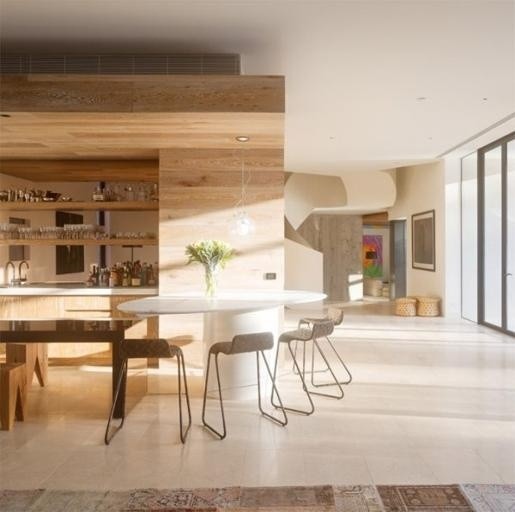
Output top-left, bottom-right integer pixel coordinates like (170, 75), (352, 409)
(186, 238), (235, 299)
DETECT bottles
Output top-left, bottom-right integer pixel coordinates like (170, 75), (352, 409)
(87, 261), (157, 288)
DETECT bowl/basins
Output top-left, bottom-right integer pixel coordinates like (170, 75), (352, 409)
(42, 192), (61, 202)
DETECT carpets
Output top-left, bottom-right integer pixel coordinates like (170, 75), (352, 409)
(0, 486), (515, 512)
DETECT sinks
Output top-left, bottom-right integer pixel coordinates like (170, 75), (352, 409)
(0, 281), (27, 288)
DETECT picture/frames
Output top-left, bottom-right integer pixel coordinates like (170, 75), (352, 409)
(412, 210), (436, 272)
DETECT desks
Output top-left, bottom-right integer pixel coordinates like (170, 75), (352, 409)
(1, 316), (160, 430)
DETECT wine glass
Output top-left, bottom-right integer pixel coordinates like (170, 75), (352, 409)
(1, 223), (109, 240)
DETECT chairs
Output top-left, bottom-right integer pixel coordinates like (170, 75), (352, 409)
(105, 307), (353, 445)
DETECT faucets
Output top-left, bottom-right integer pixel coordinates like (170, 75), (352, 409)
(6, 261), (15, 280)
(19, 261), (29, 280)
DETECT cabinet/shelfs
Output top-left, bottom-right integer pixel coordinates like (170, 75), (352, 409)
(1, 198), (159, 247)
